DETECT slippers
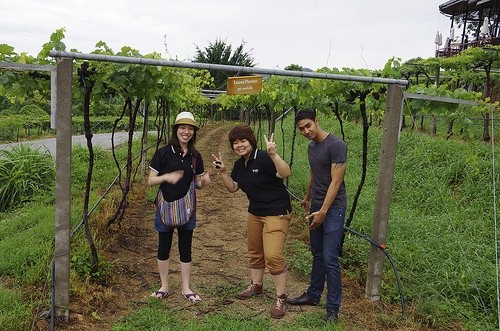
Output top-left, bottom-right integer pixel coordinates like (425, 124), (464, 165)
(181, 290), (203, 304)
(151, 288), (173, 300)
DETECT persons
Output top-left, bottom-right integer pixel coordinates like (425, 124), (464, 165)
(286, 108), (348, 327)
(211, 124), (293, 319)
(147, 112), (211, 303)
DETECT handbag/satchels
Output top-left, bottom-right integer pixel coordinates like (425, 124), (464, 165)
(156, 181), (196, 229)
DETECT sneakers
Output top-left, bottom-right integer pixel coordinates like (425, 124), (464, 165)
(271, 292), (288, 320)
(235, 280), (263, 300)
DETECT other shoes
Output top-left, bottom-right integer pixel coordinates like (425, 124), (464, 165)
(322, 308), (338, 329)
(286, 292), (319, 307)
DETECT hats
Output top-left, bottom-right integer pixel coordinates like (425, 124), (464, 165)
(171, 112), (201, 132)
